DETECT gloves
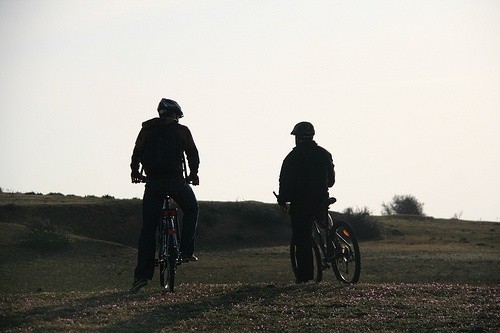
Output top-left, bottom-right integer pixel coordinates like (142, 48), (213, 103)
(131, 170), (141, 183)
(188, 172), (200, 186)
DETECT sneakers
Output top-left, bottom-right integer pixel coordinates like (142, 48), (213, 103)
(181, 255), (198, 262)
(126, 278), (147, 293)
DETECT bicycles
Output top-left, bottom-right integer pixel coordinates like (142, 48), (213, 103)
(131, 176), (194, 293)
(273, 190), (362, 284)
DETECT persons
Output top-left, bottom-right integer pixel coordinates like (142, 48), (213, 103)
(127, 98), (200, 295)
(277, 122), (335, 284)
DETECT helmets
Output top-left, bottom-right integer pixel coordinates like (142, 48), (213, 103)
(157, 98), (183, 118)
(290, 121), (315, 135)
